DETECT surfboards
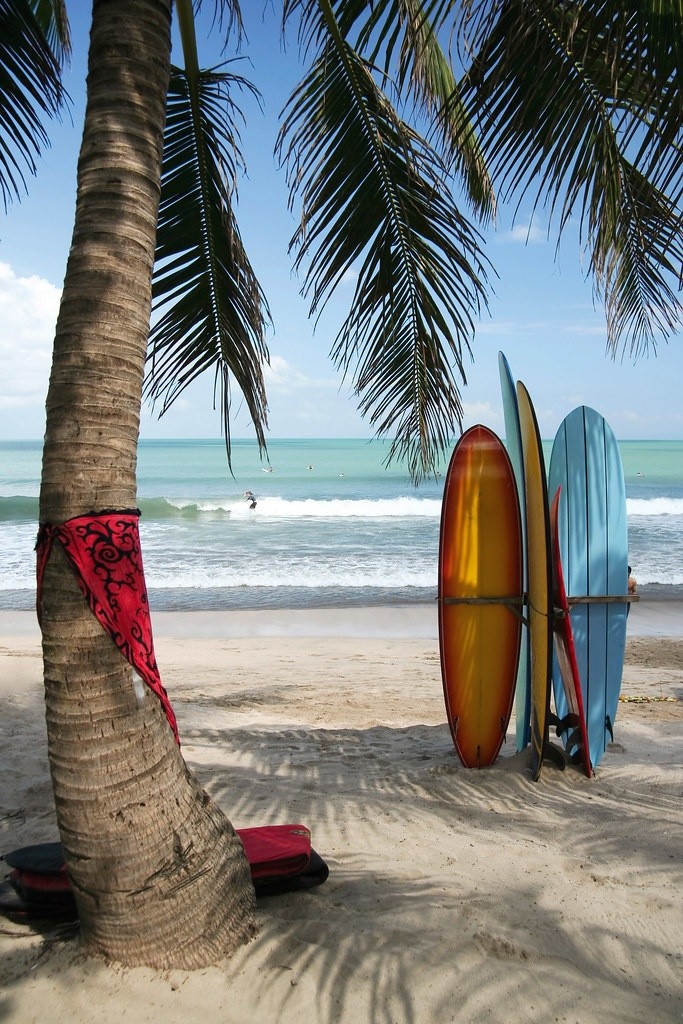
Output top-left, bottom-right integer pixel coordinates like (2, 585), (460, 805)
(544, 401), (630, 775)
(433, 420), (526, 770)
(493, 348), (555, 784)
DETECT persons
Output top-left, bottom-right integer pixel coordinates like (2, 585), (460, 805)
(627, 566), (637, 618)
(245, 492), (257, 510)
